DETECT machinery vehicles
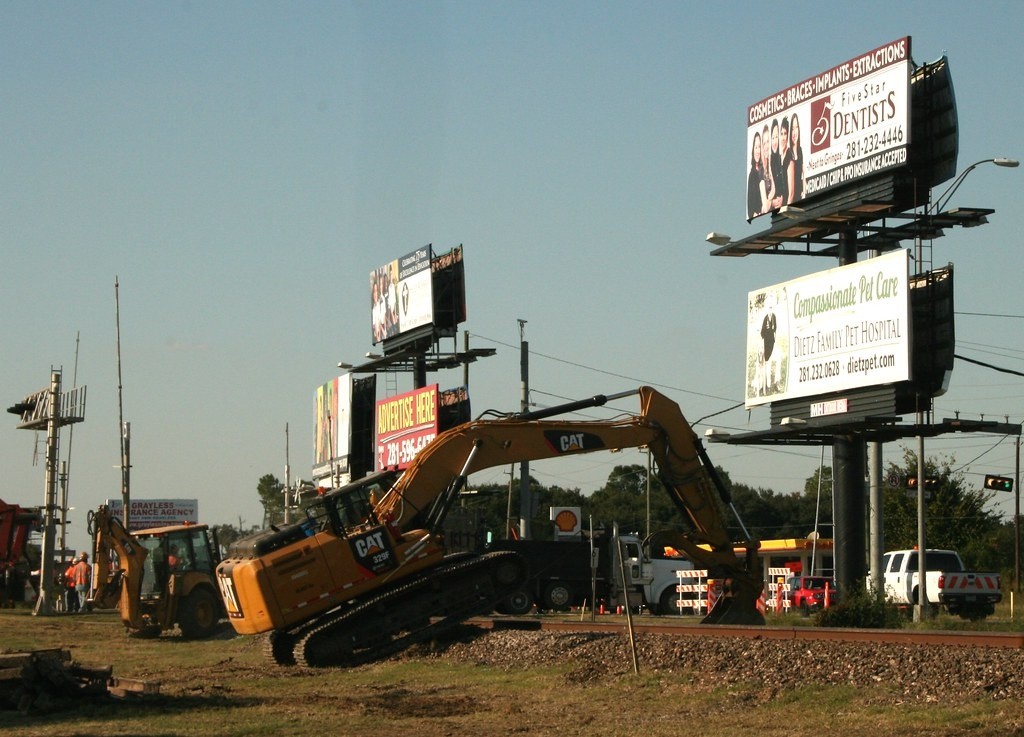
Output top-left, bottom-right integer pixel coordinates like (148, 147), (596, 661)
(215, 384), (764, 672)
(82, 504), (231, 638)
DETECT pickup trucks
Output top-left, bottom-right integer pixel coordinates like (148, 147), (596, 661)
(769, 576), (838, 618)
(864, 545), (1003, 622)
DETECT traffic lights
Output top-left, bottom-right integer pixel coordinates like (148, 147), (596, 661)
(983, 474), (1014, 493)
(906, 475), (941, 490)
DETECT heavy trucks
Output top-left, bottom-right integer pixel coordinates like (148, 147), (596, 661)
(484, 522), (694, 616)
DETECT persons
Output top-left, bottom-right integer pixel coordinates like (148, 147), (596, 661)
(748, 113), (806, 220)
(759, 300), (776, 394)
(372, 264), (399, 341)
(340, 495), (363, 524)
(28, 551), (91, 613)
(169, 544), (181, 574)
(0, 560), (17, 610)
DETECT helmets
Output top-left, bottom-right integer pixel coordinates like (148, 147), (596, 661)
(68, 557), (73, 563)
(80, 552), (89, 561)
(71, 558), (79, 564)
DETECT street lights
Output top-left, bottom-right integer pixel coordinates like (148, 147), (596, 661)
(60, 507), (75, 600)
(918, 157), (1020, 604)
